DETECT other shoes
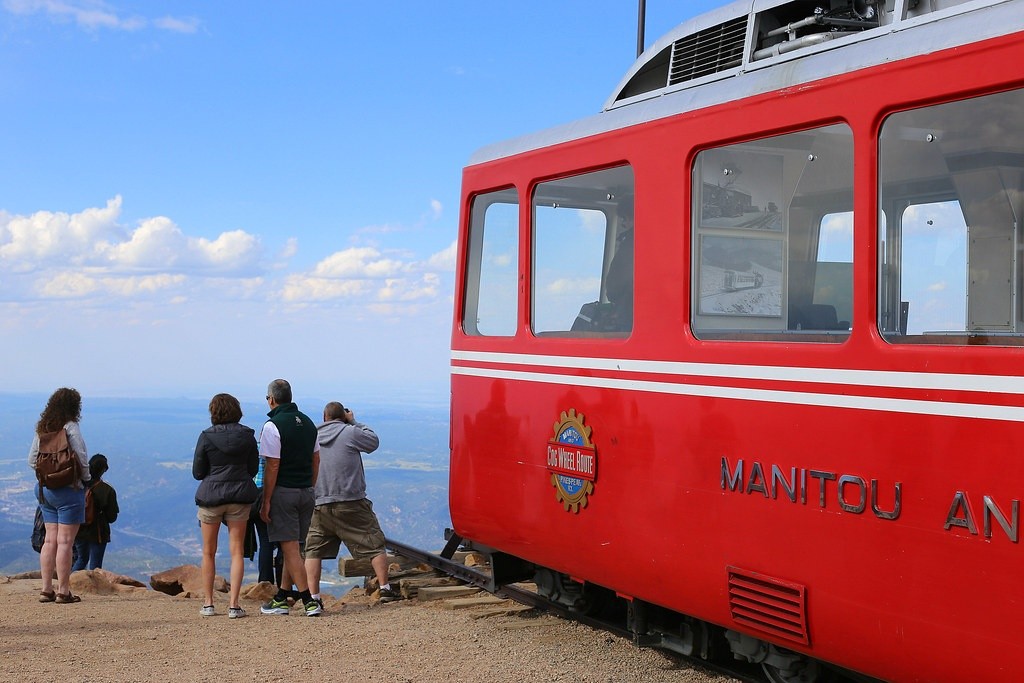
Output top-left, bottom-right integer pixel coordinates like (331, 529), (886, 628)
(200, 605), (214, 615)
(229, 607), (246, 618)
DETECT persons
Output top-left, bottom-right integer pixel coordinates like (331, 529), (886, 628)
(34, 454), (120, 573)
(192, 379), (405, 619)
(27, 386), (92, 604)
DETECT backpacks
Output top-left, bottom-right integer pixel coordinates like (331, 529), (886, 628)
(36, 429), (82, 505)
(31, 506), (46, 553)
(85, 480), (103, 524)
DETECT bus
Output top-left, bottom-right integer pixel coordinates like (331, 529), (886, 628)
(445, 0), (1023, 683)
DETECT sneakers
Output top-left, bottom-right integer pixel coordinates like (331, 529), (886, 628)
(380, 584), (404, 602)
(305, 600), (321, 616)
(316, 598), (323, 611)
(261, 595), (289, 613)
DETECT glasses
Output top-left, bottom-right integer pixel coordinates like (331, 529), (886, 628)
(266, 396), (274, 400)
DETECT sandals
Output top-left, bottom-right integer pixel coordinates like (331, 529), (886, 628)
(54, 591), (81, 603)
(39, 591), (56, 602)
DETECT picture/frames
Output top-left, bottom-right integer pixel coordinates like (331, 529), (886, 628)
(698, 149), (785, 232)
(697, 235), (787, 319)
